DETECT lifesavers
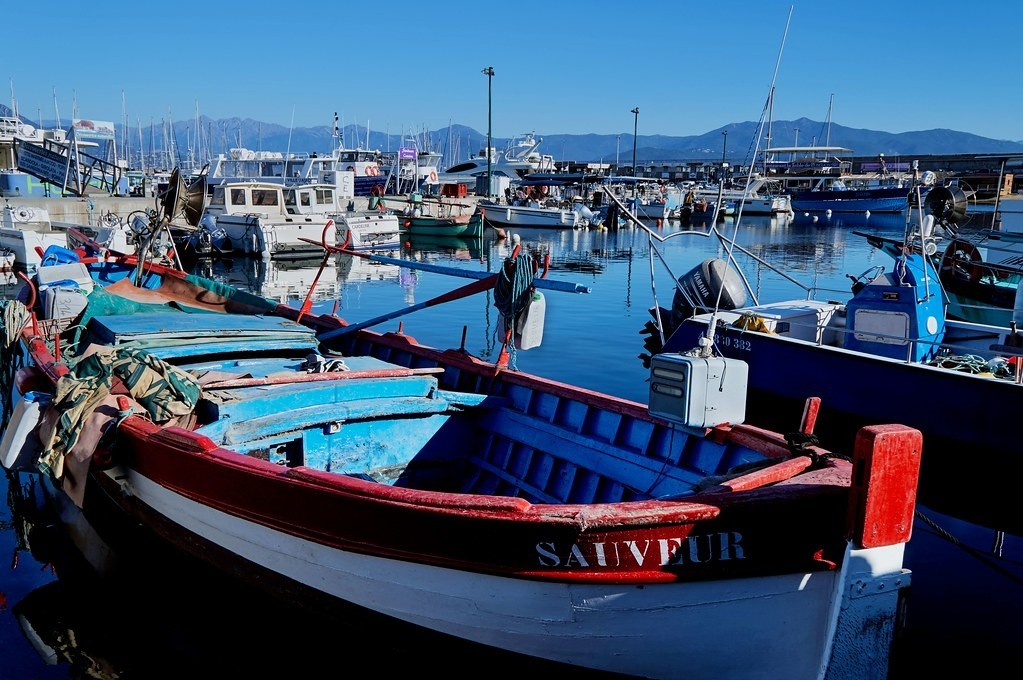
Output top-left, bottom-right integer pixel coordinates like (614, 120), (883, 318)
(430, 171), (436, 182)
(660, 185), (665, 193)
(365, 166), (374, 176)
(346, 165), (356, 177)
(943, 238), (983, 282)
(373, 165), (379, 176)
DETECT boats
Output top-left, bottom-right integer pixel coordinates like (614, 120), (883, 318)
(0, 90), (1023, 259)
(0, 225), (924, 680)
(852, 157), (1023, 333)
(605, 0), (1023, 538)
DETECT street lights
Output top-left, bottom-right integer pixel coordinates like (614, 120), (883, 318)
(481, 65), (496, 197)
(627, 105), (642, 194)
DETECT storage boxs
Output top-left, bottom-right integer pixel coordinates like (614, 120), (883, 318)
(36, 262), (94, 298)
